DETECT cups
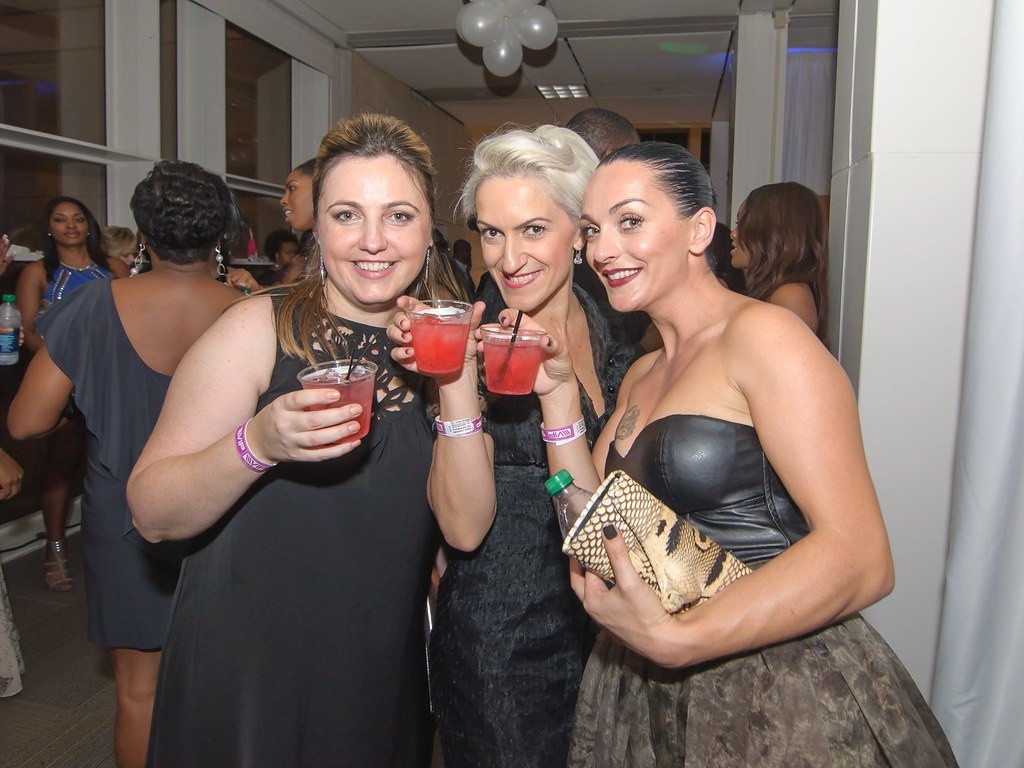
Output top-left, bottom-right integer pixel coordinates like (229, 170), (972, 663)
(405, 299), (473, 375)
(296, 359), (377, 443)
(480, 326), (549, 396)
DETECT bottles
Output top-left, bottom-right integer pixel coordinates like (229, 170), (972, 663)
(544, 469), (596, 541)
(0, 294), (21, 365)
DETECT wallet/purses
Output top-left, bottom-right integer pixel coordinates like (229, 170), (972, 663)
(562, 471), (753, 615)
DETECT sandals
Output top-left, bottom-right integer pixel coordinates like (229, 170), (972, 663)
(43, 537), (74, 593)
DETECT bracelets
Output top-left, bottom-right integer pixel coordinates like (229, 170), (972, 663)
(540, 414), (587, 446)
(431, 412), (486, 437)
(235, 418), (279, 473)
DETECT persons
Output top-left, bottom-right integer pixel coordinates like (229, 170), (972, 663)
(17, 196), (131, 591)
(474, 141), (963, 768)
(101, 108), (829, 401)
(7, 159), (245, 768)
(0, 234), (26, 698)
(125, 114), (646, 768)
